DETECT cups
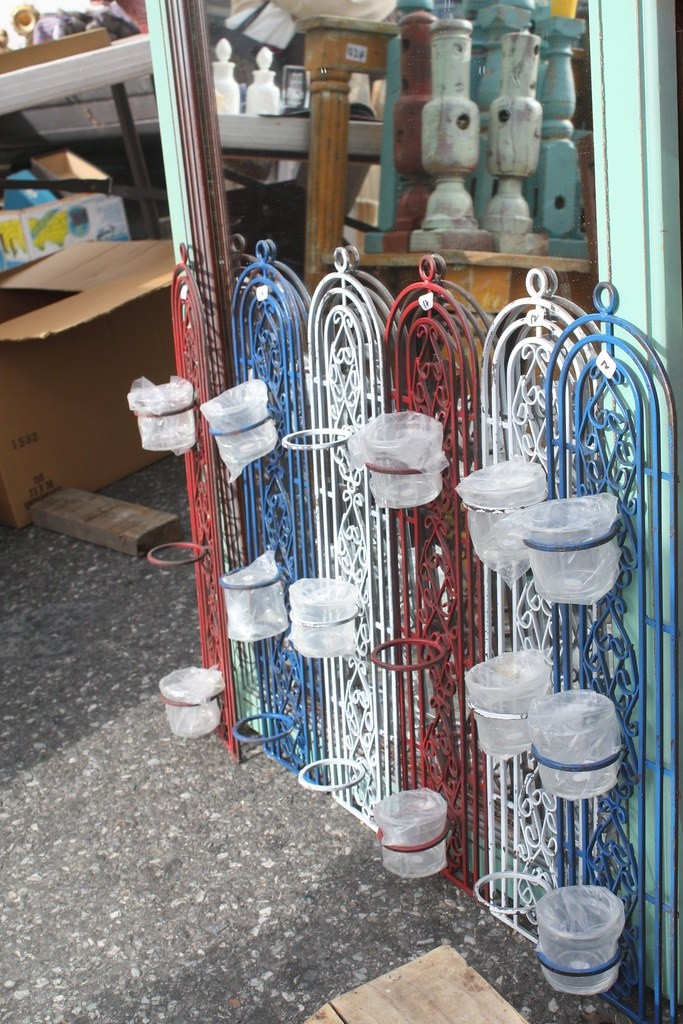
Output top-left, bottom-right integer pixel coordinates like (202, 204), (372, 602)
(220, 554), (287, 643)
(511, 497), (619, 605)
(358, 412), (443, 508)
(455, 459), (549, 572)
(159, 667), (222, 739)
(287, 577), (359, 659)
(373, 788), (448, 878)
(535, 885), (624, 996)
(464, 649), (552, 759)
(528, 689), (622, 800)
(134, 381), (197, 450)
(201, 381), (279, 467)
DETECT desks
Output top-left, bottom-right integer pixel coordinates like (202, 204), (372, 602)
(0, 34), (384, 277)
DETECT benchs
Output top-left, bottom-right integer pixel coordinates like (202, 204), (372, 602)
(299, 16), (598, 375)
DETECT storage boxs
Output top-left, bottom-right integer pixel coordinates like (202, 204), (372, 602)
(0, 194), (131, 263)
(304, 944), (530, 1023)
(31, 148), (112, 200)
(4, 170), (59, 212)
(0, 241), (176, 530)
